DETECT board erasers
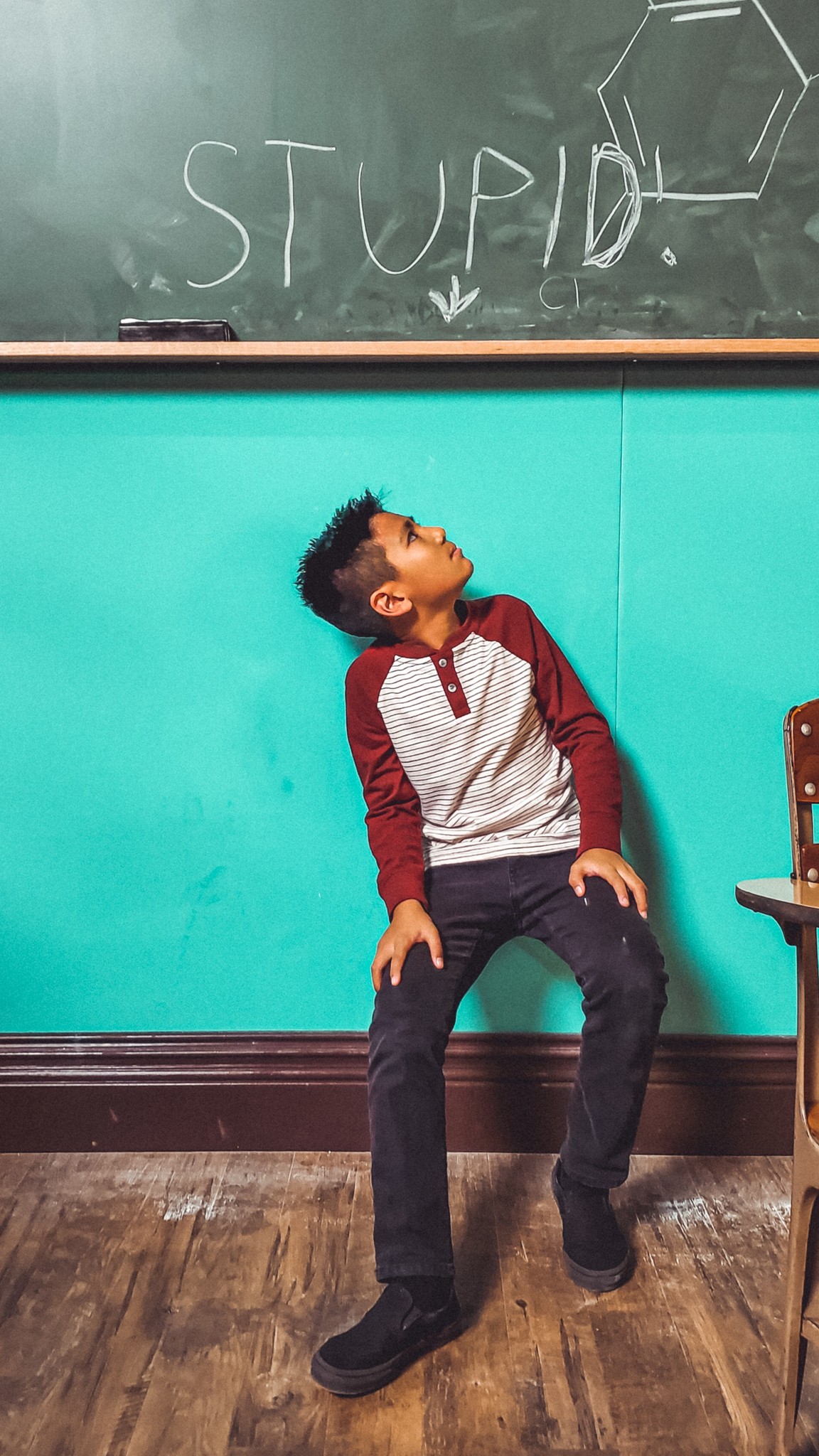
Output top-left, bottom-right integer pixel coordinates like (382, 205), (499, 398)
(119, 317), (229, 343)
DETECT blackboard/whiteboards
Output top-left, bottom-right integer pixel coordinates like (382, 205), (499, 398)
(0, 0), (817, 373)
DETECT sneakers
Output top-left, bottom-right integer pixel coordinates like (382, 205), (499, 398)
(551, 1158), (637, 1292)
(310, 1281), (466, 1395)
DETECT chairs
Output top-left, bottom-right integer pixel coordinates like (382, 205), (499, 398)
(734, 699), (819, 1455)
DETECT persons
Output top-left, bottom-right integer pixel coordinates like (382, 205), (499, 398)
(292, 488), (672, 1400)
